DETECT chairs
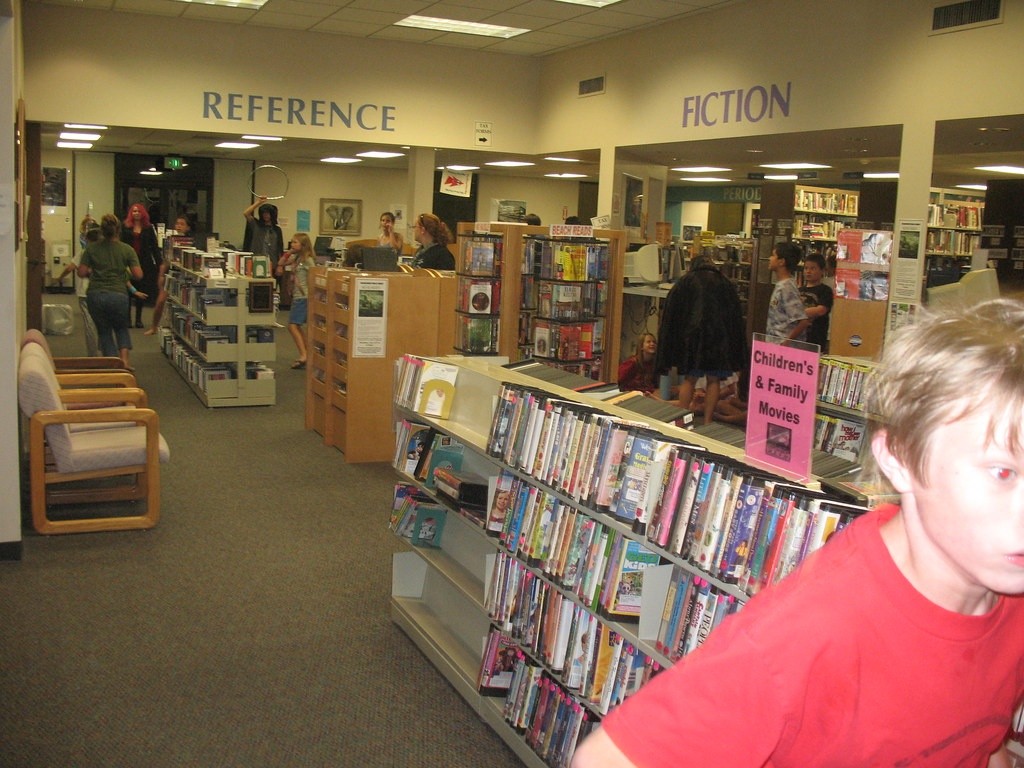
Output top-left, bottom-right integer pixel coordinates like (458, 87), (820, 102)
(16, 325), (162, 534)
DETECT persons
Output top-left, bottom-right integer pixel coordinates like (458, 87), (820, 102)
(120, 204), (163, 328)
(277, 233), (316, 368)
(765, 241), (809, 350)
(380, 211), (404, 254)
(489, 491), (510, 523)
(144, 215), (191, 335)
(407, 213), (455, 270)
(243, 195), (283, 291)
(49, 213), (149, 372)
(571, 299), (1024, 767)
(798, 253), (834, 355)
(619, 257), (750, 426)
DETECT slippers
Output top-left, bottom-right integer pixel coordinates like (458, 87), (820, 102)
(291, 359), (306, 369)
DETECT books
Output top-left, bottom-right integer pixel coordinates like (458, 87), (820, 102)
(926, 202), (984, 255)
(391, 346), (1024, 767)
(158, 233), (274, 396)
(457, 236), (609, 382)
(793, 190), (858, 239)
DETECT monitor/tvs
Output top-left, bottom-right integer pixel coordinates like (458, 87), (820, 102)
(314, 236), (333, 256)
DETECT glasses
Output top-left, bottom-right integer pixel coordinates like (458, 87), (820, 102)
(420, 213), (425, 227)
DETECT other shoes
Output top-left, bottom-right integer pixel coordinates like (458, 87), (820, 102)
(272, 322), (286, 328)
(144, 329), (157, 335)
(123, 365), (136, 372)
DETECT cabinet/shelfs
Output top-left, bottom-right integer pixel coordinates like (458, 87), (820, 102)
(302, 183), (898, 768)
(924, 186), (983, 278)
(978, 178), (1024, 288)
(166, 258), (277, 407)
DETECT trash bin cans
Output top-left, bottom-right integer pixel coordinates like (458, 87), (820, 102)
(45, 304), (71, 336)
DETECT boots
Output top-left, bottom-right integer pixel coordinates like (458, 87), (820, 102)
(135, 310), (143, 328)
(127, 307), (132, 328)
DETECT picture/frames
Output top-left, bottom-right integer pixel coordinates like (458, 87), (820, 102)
(320, 198), (362, 235)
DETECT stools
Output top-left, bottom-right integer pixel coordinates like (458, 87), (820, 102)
(42, 303), (74, 336)
